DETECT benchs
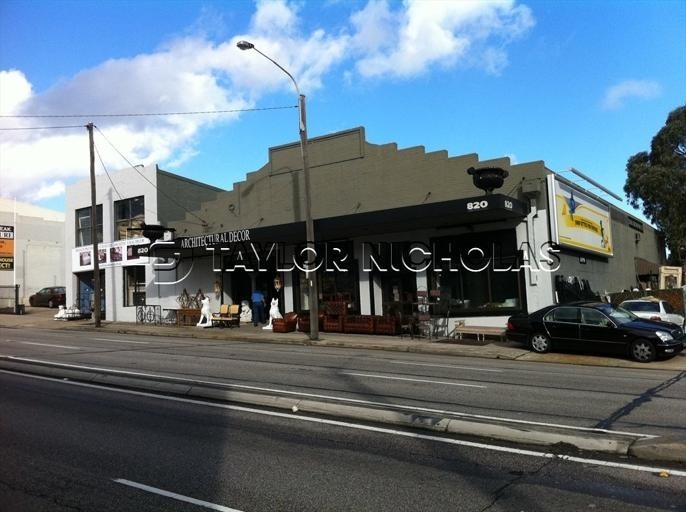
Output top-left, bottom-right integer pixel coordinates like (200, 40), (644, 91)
(455, 325), (507, 343)
(323, 315), (400, 335)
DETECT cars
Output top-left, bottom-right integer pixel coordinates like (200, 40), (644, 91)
(506, 300), (686, 362)
(29, 287), (65, 308)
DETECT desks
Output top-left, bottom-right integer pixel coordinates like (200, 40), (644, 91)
(176, 309), (200, 326)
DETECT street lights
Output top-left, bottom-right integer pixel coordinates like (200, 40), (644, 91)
(236, 41), (320, 338)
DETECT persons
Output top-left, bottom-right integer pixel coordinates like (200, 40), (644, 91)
(251, 286), (267, 327)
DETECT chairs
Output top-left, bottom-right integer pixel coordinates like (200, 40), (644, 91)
(273, 312), (297, 333)
(211, 304), (229, 328)
(399, 310), (450, 341)
(221, 305), (241, 328)
